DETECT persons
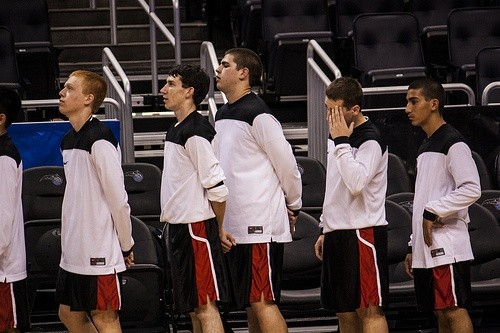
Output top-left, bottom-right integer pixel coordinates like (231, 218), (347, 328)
(55, 70), (136, 333)
(212, 47), (304, 333)
(403, 77), (482, 333)
(159, 64), (237, 333)
(0, 87), (32, 333)
(314, 77), (390, 333)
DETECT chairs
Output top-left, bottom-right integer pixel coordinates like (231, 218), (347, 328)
(0, 0), (500, 333)
